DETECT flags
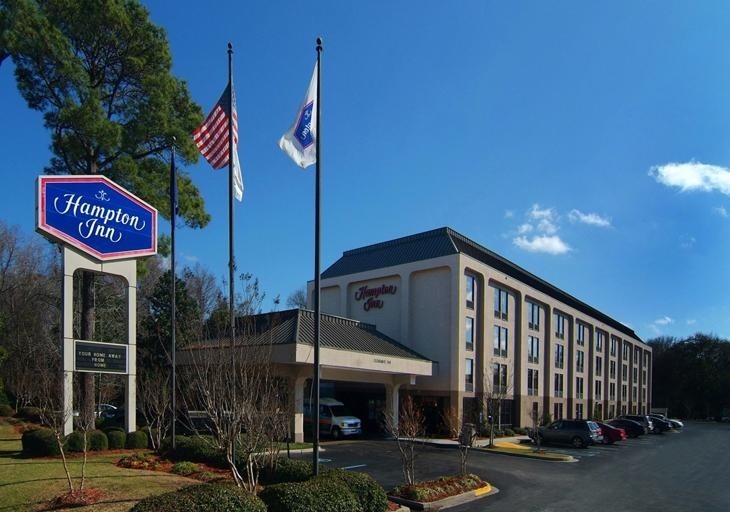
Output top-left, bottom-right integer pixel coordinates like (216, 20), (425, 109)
(192, 81), (244, 202)
(277, 63), (318, 169)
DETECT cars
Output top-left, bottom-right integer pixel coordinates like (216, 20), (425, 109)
(650, 413), (684, 429)
(70, 400), (120, 422)
(592, 420), (626, 446)
(718, 413), (730, 423)
(609, 419), (644, 439)
(650, 417), (671, 433)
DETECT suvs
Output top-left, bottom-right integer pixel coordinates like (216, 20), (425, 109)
(528, 416), (604, 450)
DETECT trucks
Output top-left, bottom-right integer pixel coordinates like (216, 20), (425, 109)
(618, 414), (654, 434)
(302, 396), (363, 440)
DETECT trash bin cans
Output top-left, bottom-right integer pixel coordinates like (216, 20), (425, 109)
(463, 423), (473, 445)
